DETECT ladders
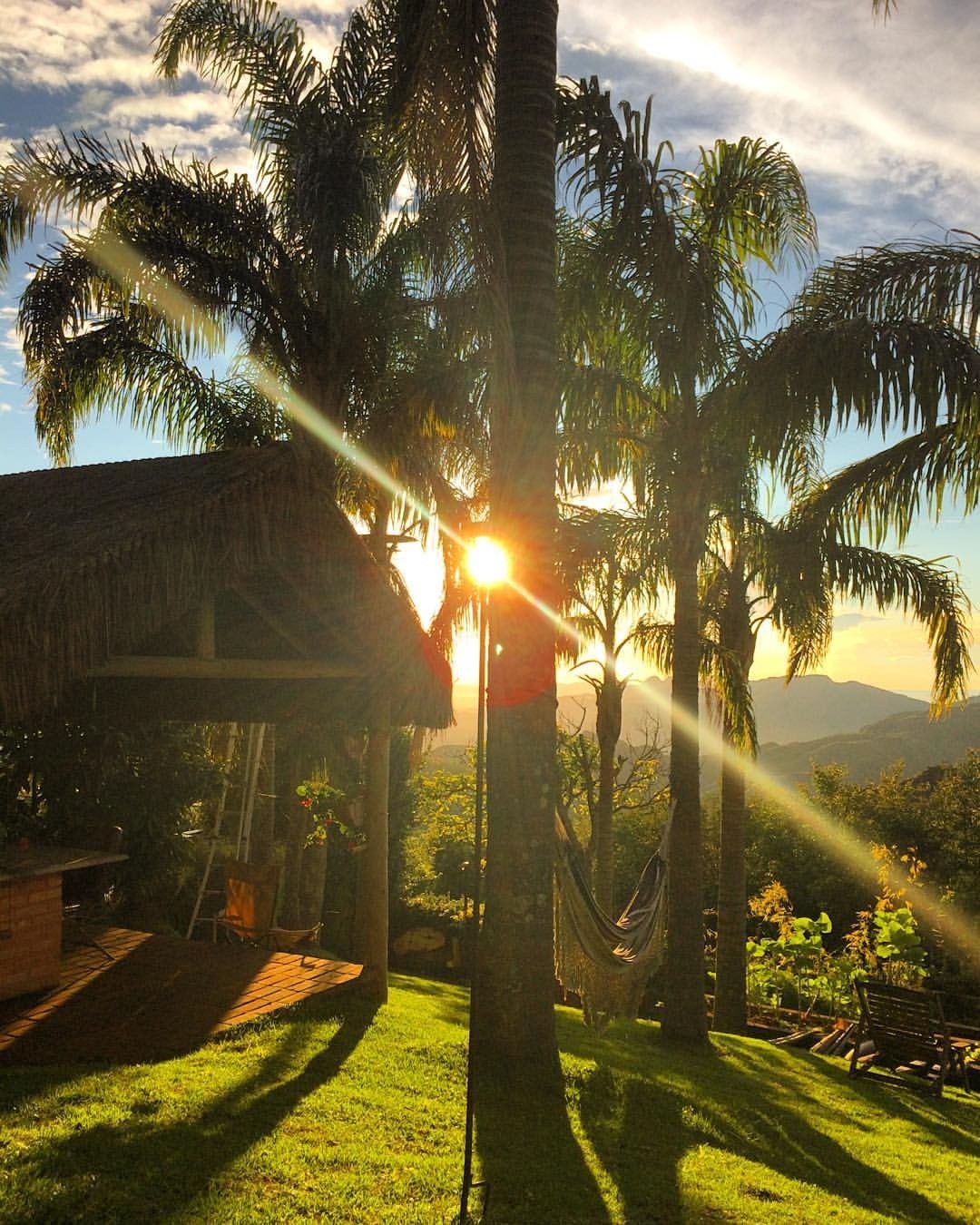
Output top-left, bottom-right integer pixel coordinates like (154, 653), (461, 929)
(185, 724), (266, 936)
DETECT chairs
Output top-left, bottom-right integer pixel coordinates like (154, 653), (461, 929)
(849, 977), (971, 1098)
(62, 819), (124, 962)
(219, 859), (324, 966)
